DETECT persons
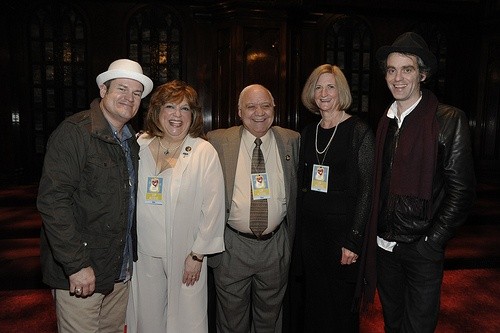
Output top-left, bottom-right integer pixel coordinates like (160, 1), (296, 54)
(282, 64), (376, 333)
(206, 84), (302, 333)
(123, 80), (225, 333)
(35, 59), (154, 333)
(377, 33), (474, 333)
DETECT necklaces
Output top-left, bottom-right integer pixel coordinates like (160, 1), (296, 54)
(315, 110), (345, 154)
(158, 136), (188, 155)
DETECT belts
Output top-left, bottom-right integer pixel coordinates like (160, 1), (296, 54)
(225, 218), (285, 240)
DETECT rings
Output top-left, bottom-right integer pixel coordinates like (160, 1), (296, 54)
(192, 275), (196, 279)
(75, 288), (82, 295)
(352, 259), (356, 263)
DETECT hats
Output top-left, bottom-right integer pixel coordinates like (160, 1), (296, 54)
(377, 31), (439, 78)
(96, 58), (154, 99)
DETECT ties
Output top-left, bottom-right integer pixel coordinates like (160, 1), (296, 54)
(249, 138), (268, 237)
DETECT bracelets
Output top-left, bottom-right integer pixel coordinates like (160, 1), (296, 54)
(190, 252), (203, 263)
